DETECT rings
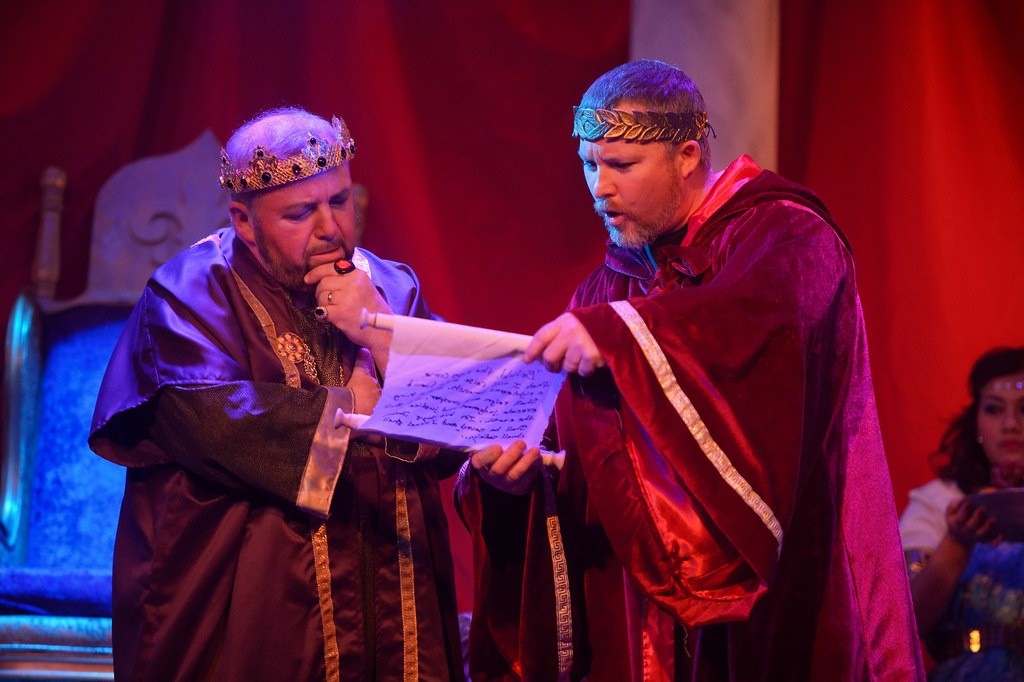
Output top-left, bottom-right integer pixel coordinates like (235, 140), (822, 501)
(328, 291), (332, 305)
(334, 260), (355, 276)
(313, 305), (328, 323)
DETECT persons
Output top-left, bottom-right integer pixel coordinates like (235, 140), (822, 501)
(901, 346), (1024, 680)
(86, 106), (470, 681)
(453, 61), (927, 682)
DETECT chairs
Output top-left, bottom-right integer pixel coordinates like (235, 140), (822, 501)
(1, 130), (236, 682)
